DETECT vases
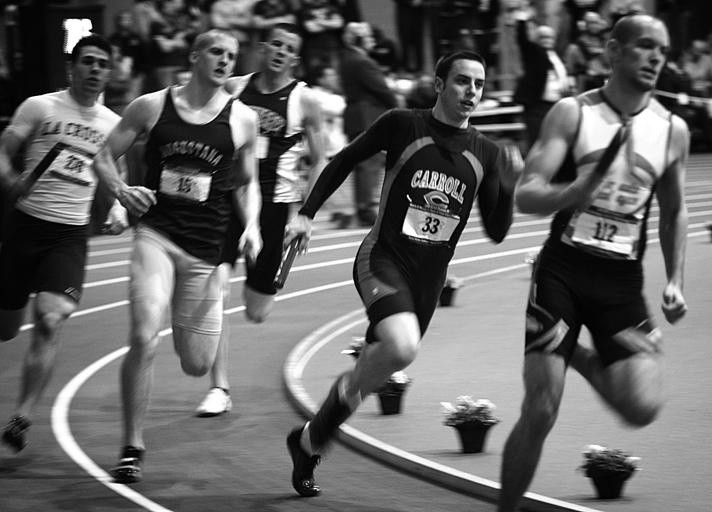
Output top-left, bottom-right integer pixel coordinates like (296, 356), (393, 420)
(452, 426), (492, 452)
(439, 287), (454, 305)
(376, 389), (407, 414)
(587, 473), (631, 498)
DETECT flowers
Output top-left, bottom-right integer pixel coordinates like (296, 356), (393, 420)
(524, 250), (540, 262)
(342, 335), (366, 356)
(578, 443), (637, 471)
(389, 370), (409, 387)
(442, 273), (467, 290)
(440, 396), (497, 425)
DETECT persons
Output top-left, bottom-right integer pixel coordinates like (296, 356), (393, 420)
(492, 15), (690, 512)
(94, 29), (264, 482)
(283, 48), (523, 503)
(1, 1), (711, 236)
(1, 35), (130, 461)
(194, 25), (328, 420)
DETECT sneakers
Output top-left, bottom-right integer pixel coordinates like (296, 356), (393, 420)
(286, 421), (322, 498)
(195, 388), (232, 416)
(114, 447), (146, 479)
(0, 417), (31, 453)
